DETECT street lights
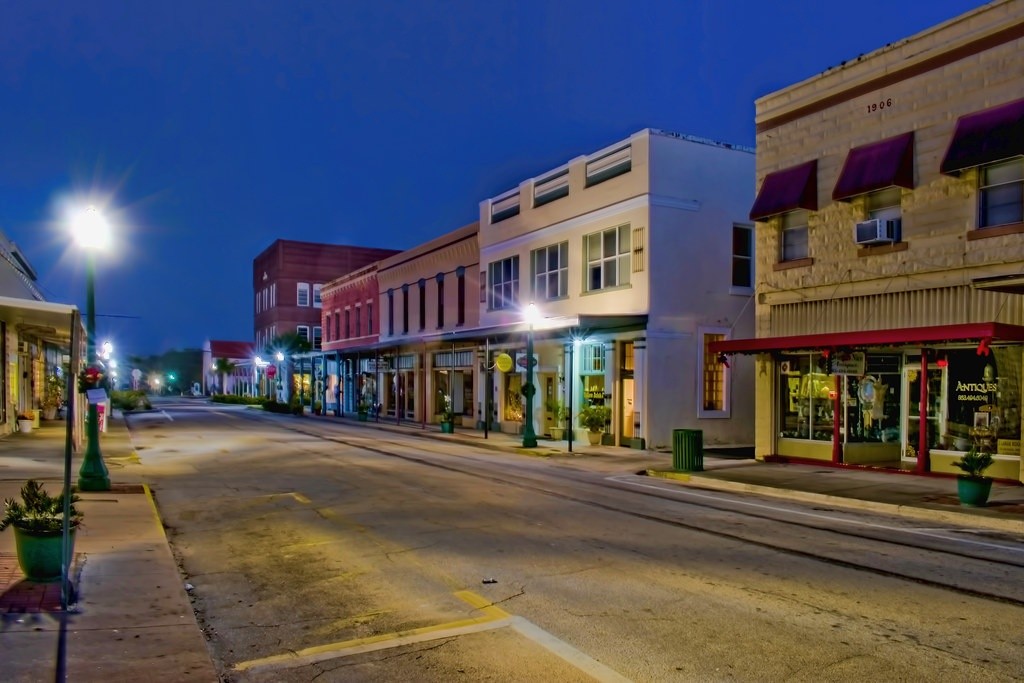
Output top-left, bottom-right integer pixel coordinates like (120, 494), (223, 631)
(276, 350), (285, 403)
(520, 303), (541, 448)
(68, 206), (117, 490)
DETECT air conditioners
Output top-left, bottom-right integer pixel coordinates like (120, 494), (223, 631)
(854, 219), (900, 246)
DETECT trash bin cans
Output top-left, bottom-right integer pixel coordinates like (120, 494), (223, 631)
(673, 428), (703, 472)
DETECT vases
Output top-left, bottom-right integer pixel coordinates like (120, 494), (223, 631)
(43, 405), (57, 420)
(18, 420), (33, 433)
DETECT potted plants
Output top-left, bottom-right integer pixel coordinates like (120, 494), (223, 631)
(440, 410), (452, 434)
(0, 477), (85, 584)
(358, 403), (370, 422)
(314, 400), (322, 416)
(546, 400), (567, 442)
(949, 447), (995, 508)
(579, 403), (611, 446)
